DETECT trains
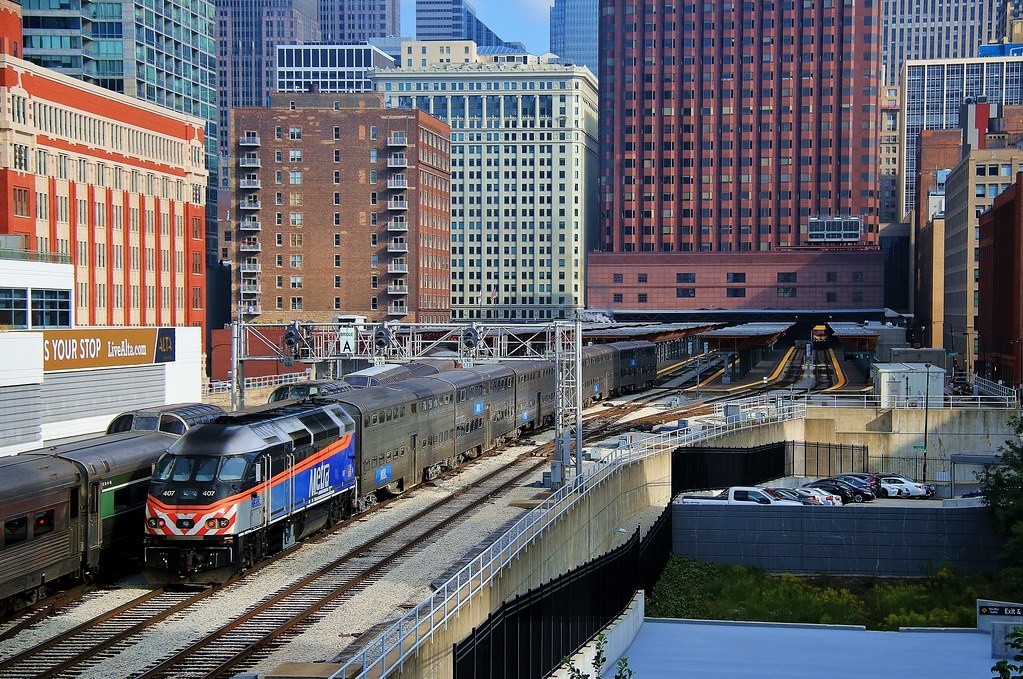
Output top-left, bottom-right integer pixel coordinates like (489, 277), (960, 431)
(0, 350), (458, 602)
(137, 339), (658, 588)
(811, 323), (831, 350)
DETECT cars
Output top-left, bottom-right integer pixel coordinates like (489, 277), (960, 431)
(746, 472), (939, 507)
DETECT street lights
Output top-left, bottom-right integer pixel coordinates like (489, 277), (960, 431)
(923, 362), (931, 483)
(976, 333), (986, 389)
(962, 332), (971, 383)
(1009, 338), (1023, 405)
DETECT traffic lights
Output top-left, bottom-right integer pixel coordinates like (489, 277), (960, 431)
(284, 327), (299, 347)
(375, 328), (391, 348)
(463, 328), (479, 349)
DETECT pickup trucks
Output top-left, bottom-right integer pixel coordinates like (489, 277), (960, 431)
(679, 487), (804, 506)
